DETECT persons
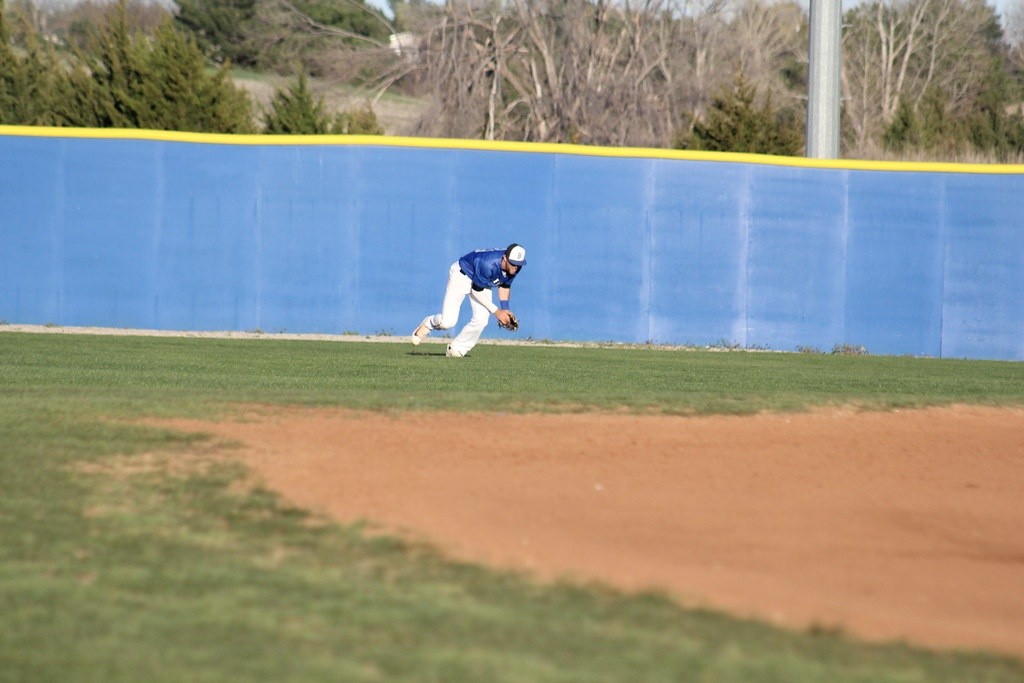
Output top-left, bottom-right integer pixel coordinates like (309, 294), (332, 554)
(412, 243), (527, 358)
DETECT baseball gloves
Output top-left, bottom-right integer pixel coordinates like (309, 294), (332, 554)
(497, 312), (519, 332)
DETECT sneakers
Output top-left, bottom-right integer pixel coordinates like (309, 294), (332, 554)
(446, 344), (462, 357)
(411, 316), (433, 345)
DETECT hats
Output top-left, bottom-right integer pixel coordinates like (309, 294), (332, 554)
(504, 243), (527, 266)
